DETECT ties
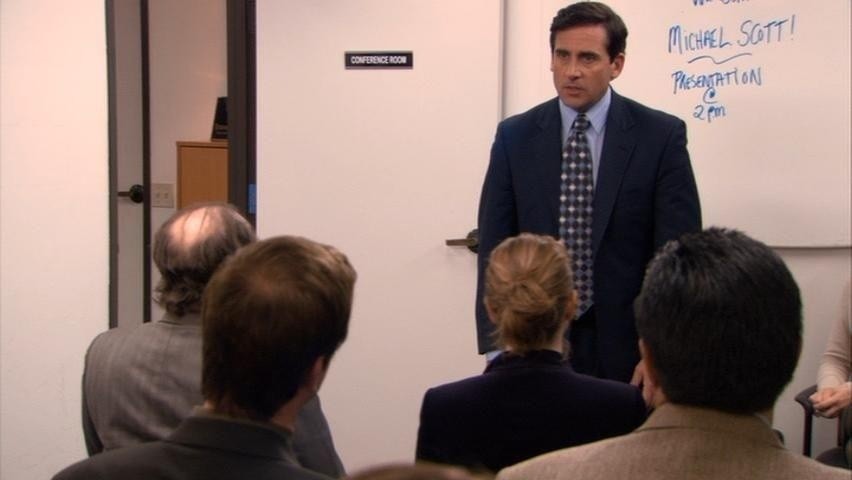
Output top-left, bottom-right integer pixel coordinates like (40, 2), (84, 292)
(557, 112), (597, 323)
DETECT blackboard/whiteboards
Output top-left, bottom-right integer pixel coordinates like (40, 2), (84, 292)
(499, 2), (852, 248)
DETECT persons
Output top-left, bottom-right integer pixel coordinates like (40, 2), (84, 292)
(80, 203), (346, 476)
(474, 1), (704, 409)
(52, 235), (356, 480)
(413, 233), (647, 475)
(496, 226), (852, 480)
(807, 265), (851, 471)
